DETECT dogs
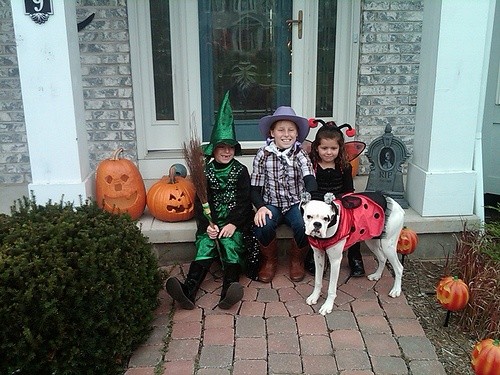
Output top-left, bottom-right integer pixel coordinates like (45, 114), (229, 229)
(300, 192), (405, 315)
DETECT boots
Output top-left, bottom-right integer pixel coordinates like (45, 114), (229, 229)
(310, 251), (325, 276)
(258, 237), (278, 282)
(166, 261), (208, 310)
(290, 238), (310, 281)
(347, 242), (366, 277)
(218, 263), (244, 309)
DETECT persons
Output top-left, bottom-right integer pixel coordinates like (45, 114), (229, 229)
(306, 125), (366, 277)
(166, 90), (250, 310)
(250, 106), (321, 282)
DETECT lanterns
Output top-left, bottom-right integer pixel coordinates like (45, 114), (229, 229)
(95, 148), (146, 221)
(146, 169), (196, 221)
(397, 226), (419, 255)
(471, 339), (500, 375)
(437, 276), (470, 311)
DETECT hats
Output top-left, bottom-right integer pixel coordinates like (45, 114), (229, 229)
(258, 106), (310, 144)
(199, 90), (242, 156)
